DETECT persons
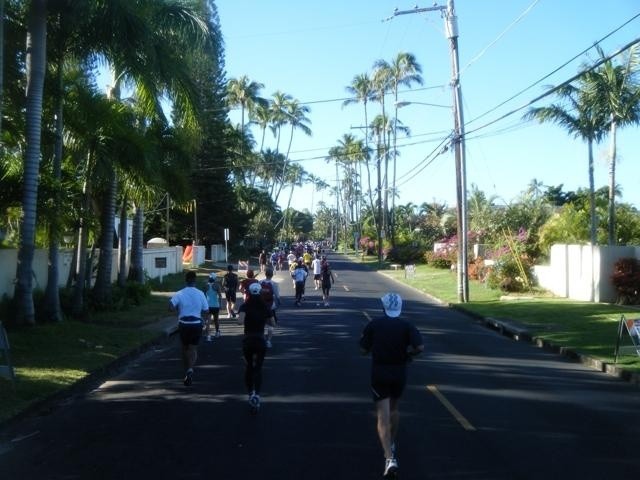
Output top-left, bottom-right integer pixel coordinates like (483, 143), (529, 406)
(168, 238), (341, 413)
(359, 292), (424, 480)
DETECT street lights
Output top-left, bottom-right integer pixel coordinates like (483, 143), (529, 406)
(339, 157), (387, 268)
(394, 98), (468, 300)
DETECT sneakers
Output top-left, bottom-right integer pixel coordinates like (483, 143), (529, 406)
(383, 441), (398, 479)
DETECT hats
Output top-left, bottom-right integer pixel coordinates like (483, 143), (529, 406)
(381, 292), (402, 318)
(209, 272), (216, 280)
(248, 283), (262, 295)
